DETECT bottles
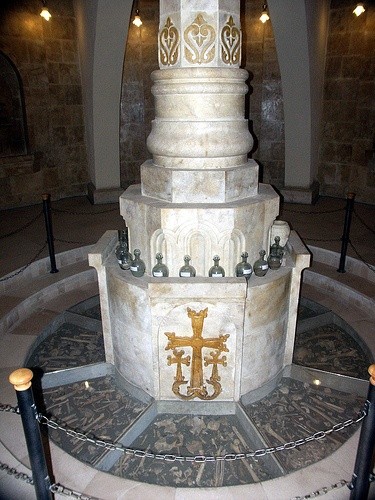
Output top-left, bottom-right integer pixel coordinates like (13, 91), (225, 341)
(267, 244), (282, 270)
(179, 255), (196, 277)
(114, 229), (134, 270)
(253, 250), (269, 277)
(209, 255), (225, 277)
(271, 220), (291, 247)
(270, 236), (284, 259)
(152, 253), (169, 277)
(129, 249), (146, 278)
(235, 251), (252, 280)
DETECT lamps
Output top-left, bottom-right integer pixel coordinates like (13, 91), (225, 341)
(133, 0), (143, 27)
(39, 0), (52, 22)
(259, 0), (271, 23)
(353, 0), (366, 16)
